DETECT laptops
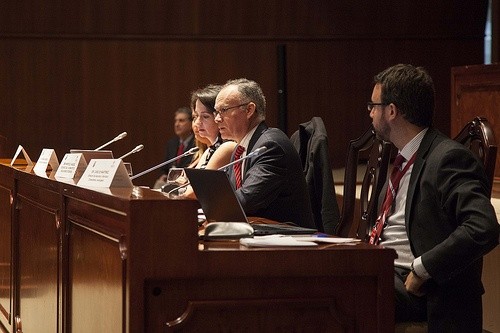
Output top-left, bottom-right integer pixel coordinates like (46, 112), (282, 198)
(70, 150), (114, 166)
(184, 167), (319, 235)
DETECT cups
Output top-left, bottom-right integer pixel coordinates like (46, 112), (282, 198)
(124, 163), (132, 181)
(167, 168), (188, 199)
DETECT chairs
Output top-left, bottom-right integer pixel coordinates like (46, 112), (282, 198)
(288, 117), (497, 333)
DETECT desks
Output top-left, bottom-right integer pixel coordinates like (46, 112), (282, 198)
(0, 159), (398, 333)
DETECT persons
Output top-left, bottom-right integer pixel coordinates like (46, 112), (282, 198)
(366, 64), (500, 333)
(154, 79), (316, 229)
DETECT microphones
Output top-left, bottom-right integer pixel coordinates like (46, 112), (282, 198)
(120, 145), (144, 159)
(169, 146), (267, 197)
(130, 147), (199, 180)
(96, 132), (128, 151)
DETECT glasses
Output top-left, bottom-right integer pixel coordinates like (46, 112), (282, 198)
(213, 102), (249, 117)
(367, 102), (389, 110)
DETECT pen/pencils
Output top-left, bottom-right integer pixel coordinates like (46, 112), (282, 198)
(279, 234), (331, 237)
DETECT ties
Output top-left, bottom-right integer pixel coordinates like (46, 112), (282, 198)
(369, 154), (417, 246)
(233, 146), (245, 191)
(176, 143), (185, 163)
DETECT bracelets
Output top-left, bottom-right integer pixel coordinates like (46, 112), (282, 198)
(411, 262), (422, 281)
